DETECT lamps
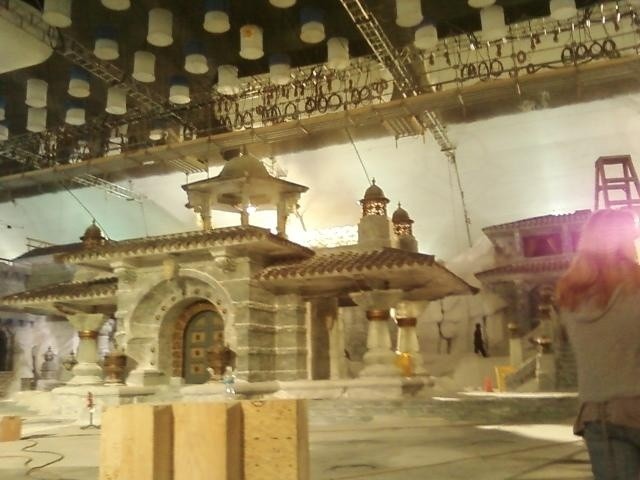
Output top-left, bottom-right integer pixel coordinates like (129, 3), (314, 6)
(1, 1), (351, 164)
(393, 1), (578, 49)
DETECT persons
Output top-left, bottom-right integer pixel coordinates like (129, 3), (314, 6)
(551, 209), (640, 479)
(472, 324), (489, 357)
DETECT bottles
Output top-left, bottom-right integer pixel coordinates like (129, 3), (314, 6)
(221, 365), (238, 400)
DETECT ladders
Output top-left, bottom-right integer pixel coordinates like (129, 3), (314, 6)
(594, 154), (640, 211)
